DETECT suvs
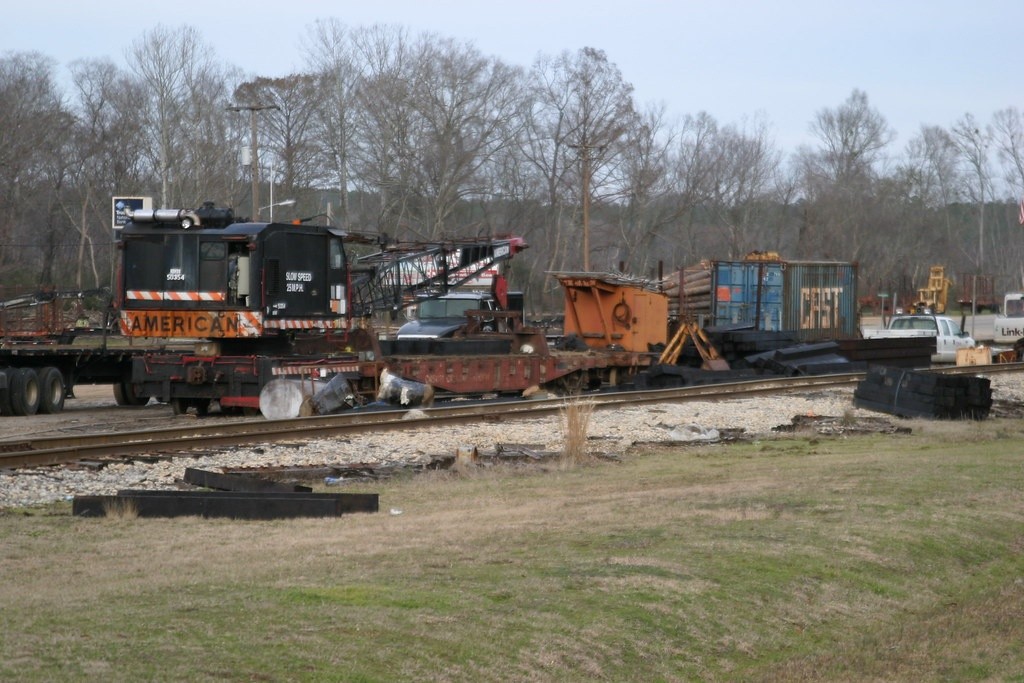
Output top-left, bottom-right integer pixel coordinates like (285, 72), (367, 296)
(393, 289), (524, 340)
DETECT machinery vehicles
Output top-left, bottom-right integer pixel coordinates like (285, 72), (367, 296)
(907, 264), (954, 315)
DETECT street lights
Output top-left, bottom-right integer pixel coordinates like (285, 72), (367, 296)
(251, 199), (298, 225)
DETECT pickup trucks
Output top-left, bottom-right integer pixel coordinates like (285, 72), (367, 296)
(862, 315), (975, 365)
